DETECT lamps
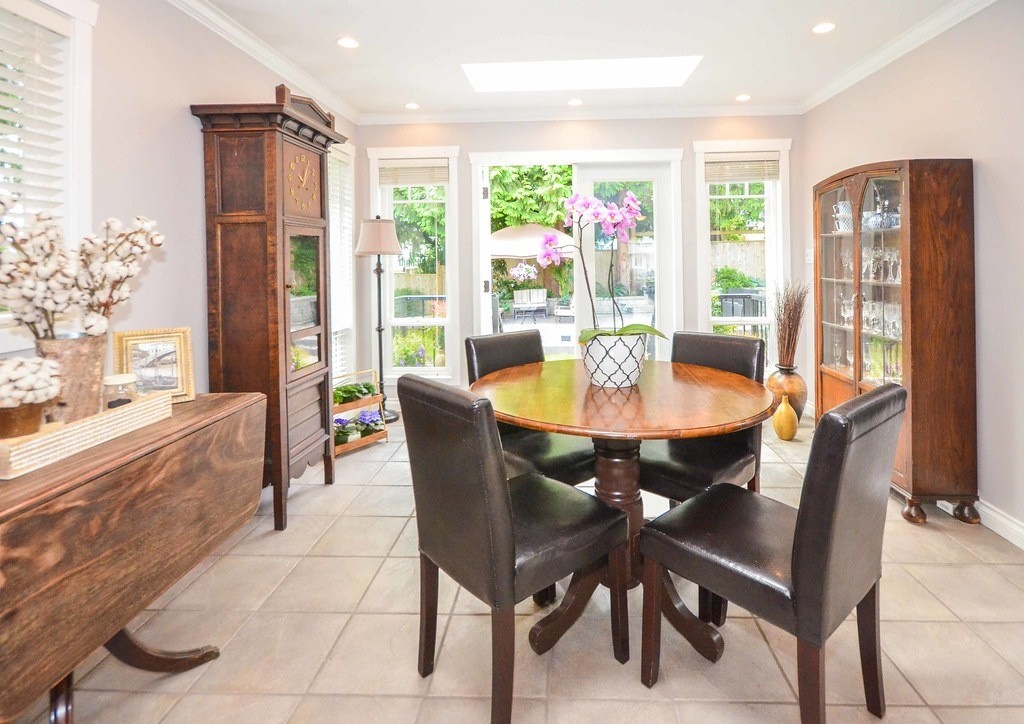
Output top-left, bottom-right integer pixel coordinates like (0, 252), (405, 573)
(354, 214), (404, 424)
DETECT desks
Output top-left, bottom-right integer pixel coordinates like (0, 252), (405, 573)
(0, 393), (265, 724)
(472, 361), (775, 662)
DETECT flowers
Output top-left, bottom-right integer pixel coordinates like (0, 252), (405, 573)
(536, 191), (668, 338)
(333, 418), (353, 439)
(354, 411), (385, 432)
(0, 357), (61, 408)
(0, 193), (163, 339)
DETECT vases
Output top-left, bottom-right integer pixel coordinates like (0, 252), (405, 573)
(774, 395), (797, 440)
(583, 334), (644, 389)
(360, 426), (374, 437)
(0, 404), (42, 438)
(767, 364), (807, 423)
(34, 334), (108, 426)
(335, 435), (348, 445)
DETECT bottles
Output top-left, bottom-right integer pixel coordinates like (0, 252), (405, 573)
(831, 200), (901, 231)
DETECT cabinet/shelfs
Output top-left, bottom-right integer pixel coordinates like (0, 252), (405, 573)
(812, 159), (983, 526)
(332, 393), (387, 455)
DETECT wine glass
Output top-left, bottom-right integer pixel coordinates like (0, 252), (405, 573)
(832, 242), (903, 386)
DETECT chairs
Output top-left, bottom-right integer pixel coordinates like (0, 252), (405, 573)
(513, 288), (574, 323)
(636, 384), (907, 723)
(469, 330), (598, 487)
(399, 375), (628, 724)
(634, 332), (765, 507)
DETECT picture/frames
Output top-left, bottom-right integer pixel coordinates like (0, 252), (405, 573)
(112, 326), (196, 405)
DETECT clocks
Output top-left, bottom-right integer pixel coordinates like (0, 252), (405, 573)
(190, 87), (348, 530)
